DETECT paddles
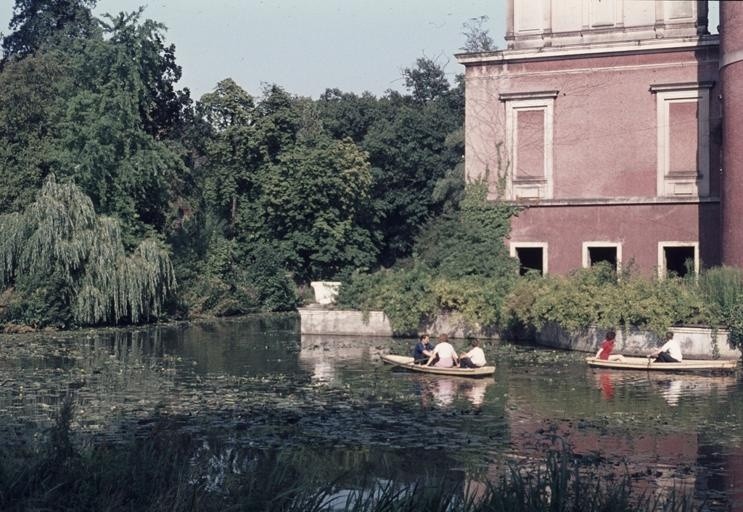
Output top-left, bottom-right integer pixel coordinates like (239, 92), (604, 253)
(377, 357), (430, 372)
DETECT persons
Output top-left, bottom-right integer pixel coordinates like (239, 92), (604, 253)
(425, 332), (460, 369)
(593, 331), (626, 362)
(457, 338), (487, 369)
(412, 333), (438, 366)
(647, 331), (683, 363)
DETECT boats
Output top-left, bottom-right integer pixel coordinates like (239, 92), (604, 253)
(379, 354), (496, 377)
(585, 356), (737, 372)
